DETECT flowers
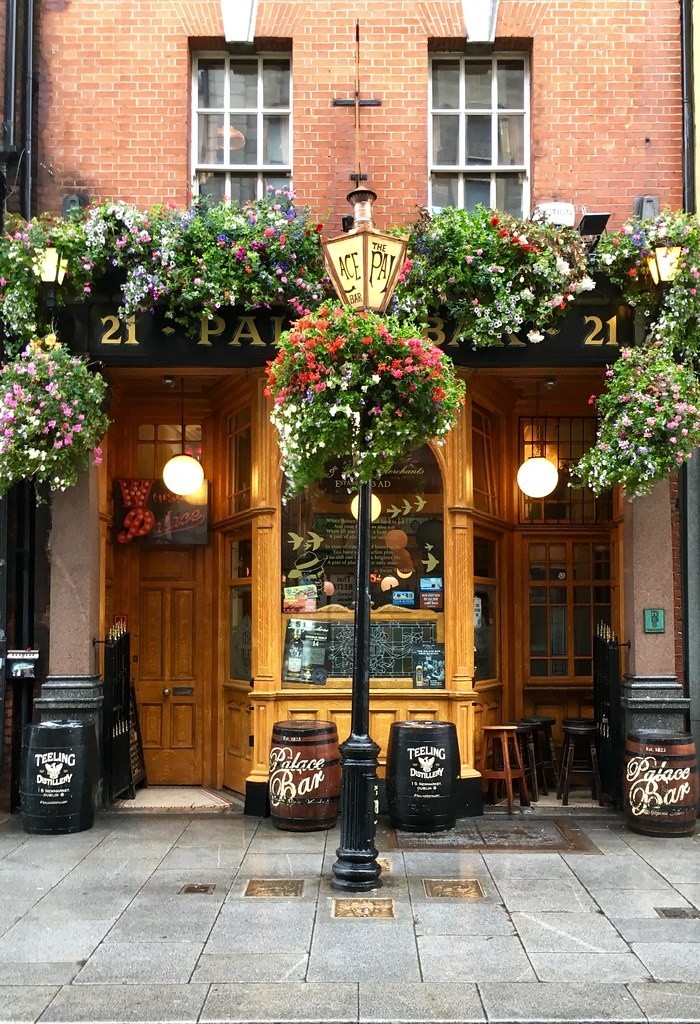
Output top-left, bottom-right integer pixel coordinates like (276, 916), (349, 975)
(0, 186), (700, 511)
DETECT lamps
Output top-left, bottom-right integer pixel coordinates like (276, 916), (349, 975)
(215, 123), (245, 151)
(162, 378), (204, 496)
(644, 236), (687, 310)
(516, 381), (558, 498)
(574, 213), (611, 252)
(34, 244), (72, 329)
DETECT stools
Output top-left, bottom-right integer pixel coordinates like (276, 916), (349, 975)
(481, 716), (604, 811)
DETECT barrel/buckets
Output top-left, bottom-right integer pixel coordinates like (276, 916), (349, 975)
(20, 719), (98, 834)
(269, 719), (341, 831)
(622, 729), (700, 838)
(386, 721), (461, 833)
